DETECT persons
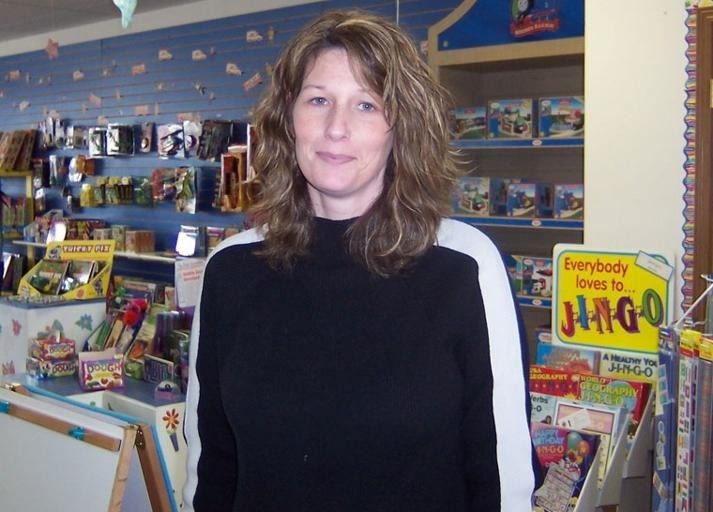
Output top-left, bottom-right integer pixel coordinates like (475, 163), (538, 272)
(177, 7), (543, 511)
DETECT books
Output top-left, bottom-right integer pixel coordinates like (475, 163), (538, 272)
(554, 401), (627, 445)
(527, 362), (580, 400)
(580, 373), (651, 439)
(600, 349), (659, 393)
(530, 420), (601, 512)
(530, 390), (563, 427)
(535, 341), (600, 378)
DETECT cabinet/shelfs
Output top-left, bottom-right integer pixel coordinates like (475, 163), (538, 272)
(424, 0), (584, 318)
(526, 331), (661, 511)
